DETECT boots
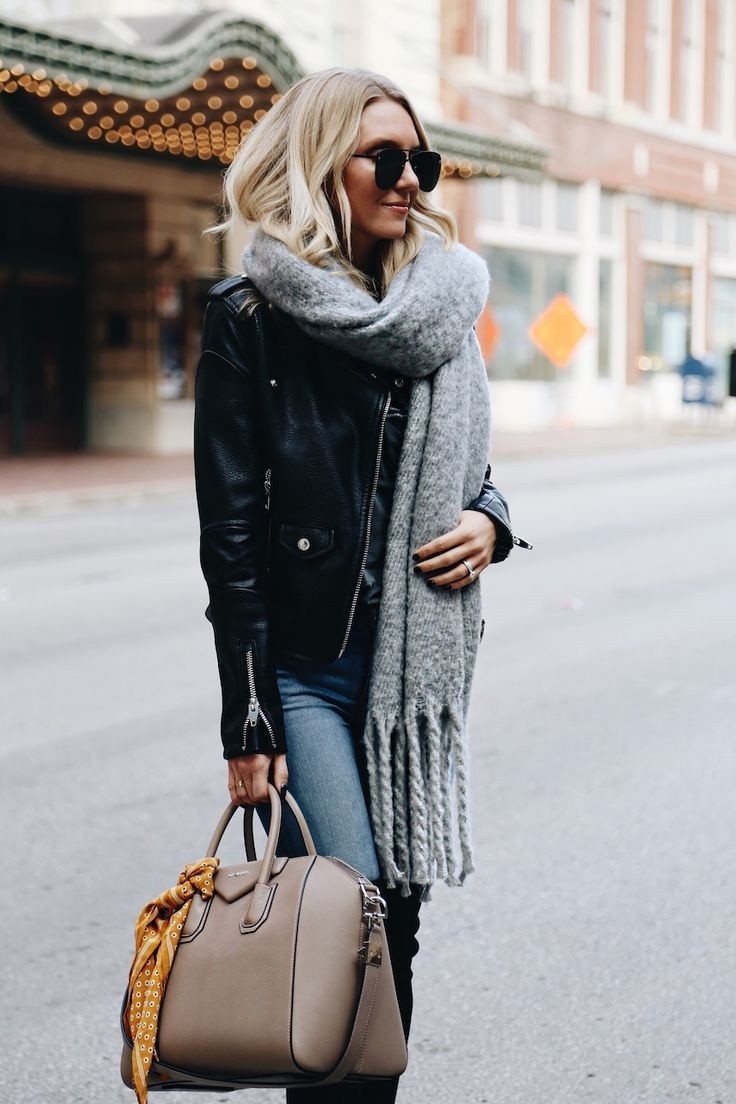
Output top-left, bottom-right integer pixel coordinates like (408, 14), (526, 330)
(286, 881), (423, 1104)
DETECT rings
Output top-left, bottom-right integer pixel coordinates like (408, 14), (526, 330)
(462, 560), (474, 576)
(236, 780), (244, 787)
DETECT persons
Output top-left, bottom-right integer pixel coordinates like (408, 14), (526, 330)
(192, 68), (512, 1104)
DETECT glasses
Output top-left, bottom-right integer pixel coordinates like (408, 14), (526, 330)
(347, 149), (442, 193)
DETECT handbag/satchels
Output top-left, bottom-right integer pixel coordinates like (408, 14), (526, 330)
(120, 784), (408, 1093)
